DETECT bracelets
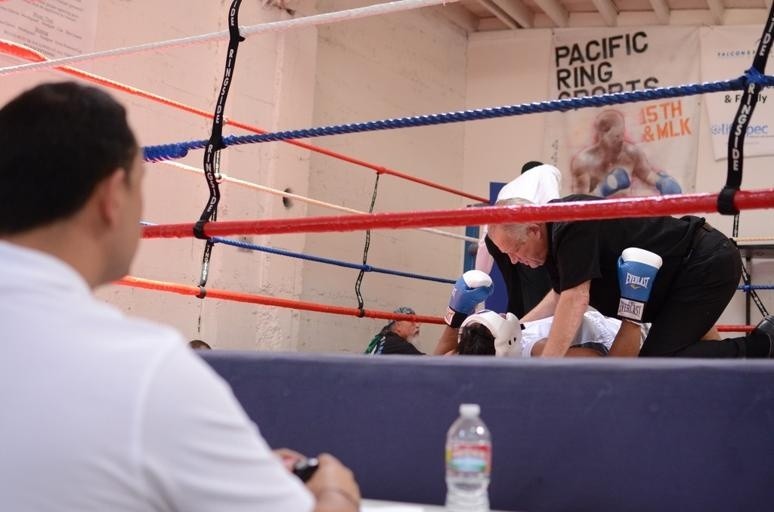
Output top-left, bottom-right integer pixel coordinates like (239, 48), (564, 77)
(315, 478), (359, 512)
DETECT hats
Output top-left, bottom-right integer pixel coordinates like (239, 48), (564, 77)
(387, 308), (415, 326)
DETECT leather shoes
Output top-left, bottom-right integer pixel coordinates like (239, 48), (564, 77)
(755, 315), (774, 358)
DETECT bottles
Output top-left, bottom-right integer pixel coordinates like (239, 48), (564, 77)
(444, 403), (492, 511)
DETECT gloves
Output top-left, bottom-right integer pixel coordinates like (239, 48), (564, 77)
(600, 167), (630, 196)
(444, 270), (494, 328)
(656, 177), (681, 195)
(617, 248), (663, 322)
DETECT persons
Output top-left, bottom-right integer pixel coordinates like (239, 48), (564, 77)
(570, 110), (686, 200)
(364, 305), (428, 354)
(486, 192), (774, 358)
(2, 81), (363, 512)
(434, 246), (724, 360)
(183, 338), (213, 353)
(498, 159), (559, 206)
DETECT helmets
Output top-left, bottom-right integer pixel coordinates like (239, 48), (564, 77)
(458, 310), (522, 358)
